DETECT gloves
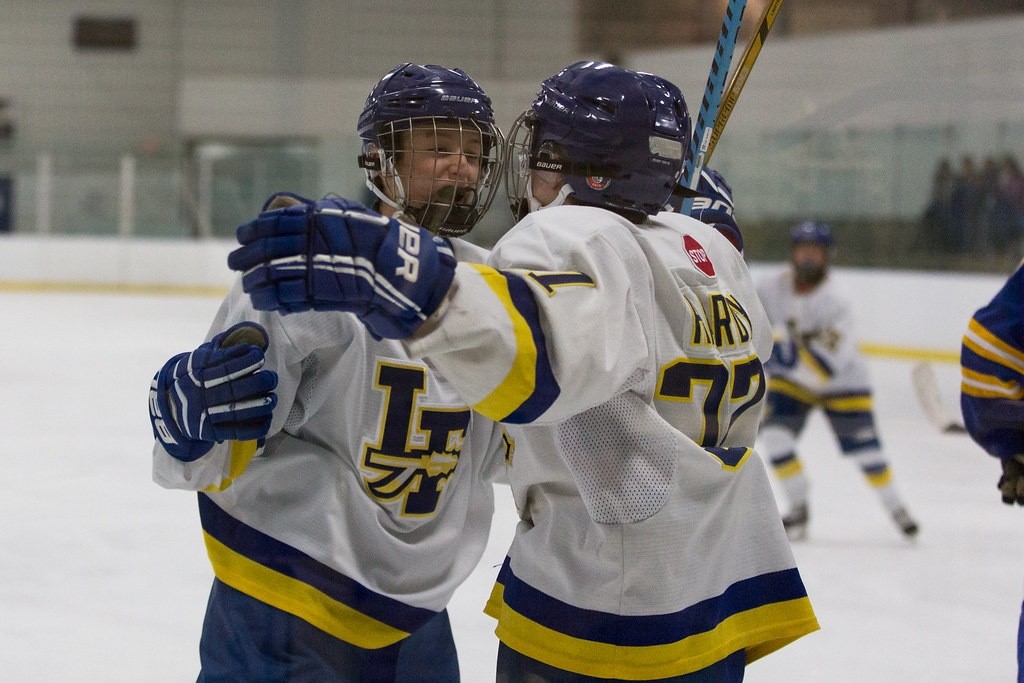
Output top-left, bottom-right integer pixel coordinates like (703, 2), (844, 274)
(678, 167), (745, 252)
(226, 192), (456, 340)
(148, 321), (278, 462)
(997, 451), (1024, 506)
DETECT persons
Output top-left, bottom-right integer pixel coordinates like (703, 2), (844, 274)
(148, 65), (518, 683)
(228, 61), (821, 683)
(750, 218), (920, 544)
(960, 260), (1024, 683)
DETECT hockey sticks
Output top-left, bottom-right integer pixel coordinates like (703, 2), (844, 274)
(672, 1), (748, 218)
(701, 0), (784, 169)
(910, 358), (968, 437)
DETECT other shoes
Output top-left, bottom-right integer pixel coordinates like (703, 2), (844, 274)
(892, 510), (918, 536)
(778, 505), (810, 539)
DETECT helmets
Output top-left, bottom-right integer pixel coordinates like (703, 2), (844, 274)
(358, 64), (507, 237)
(505, 60), (689, 223)
(789, 221), (834, 245)
